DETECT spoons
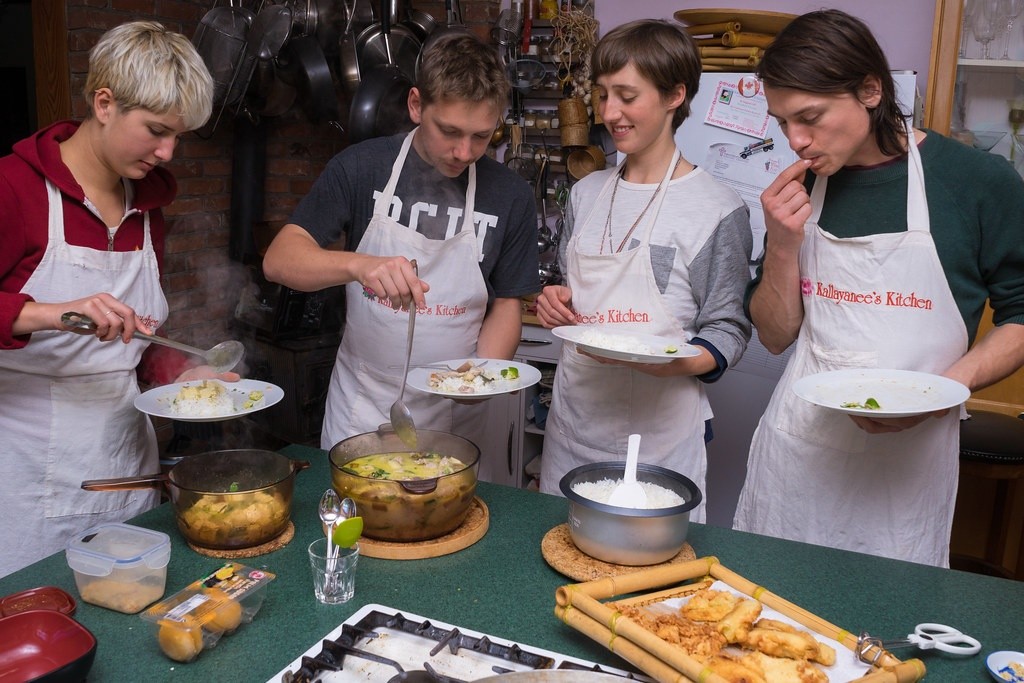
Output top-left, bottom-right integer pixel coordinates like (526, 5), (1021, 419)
(319, 489), (357, 596)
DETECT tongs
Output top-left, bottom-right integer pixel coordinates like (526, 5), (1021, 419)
(855, 623), (982, 665)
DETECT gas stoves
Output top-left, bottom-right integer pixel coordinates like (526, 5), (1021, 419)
(264, 602), (661, 683)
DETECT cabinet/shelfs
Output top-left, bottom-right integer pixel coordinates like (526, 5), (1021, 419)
(923, 0), (1024, 579)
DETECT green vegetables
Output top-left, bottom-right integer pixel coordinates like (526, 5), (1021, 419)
(365, 452), (453, 481)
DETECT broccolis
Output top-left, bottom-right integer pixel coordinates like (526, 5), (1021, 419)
(501, 367), (519, 379)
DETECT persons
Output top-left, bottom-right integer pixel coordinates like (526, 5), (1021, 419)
(538, 19), (753, 525)
(263, 32), (544, 487)
(0, 20), (241, 576)
(732, 10), (1024, 572)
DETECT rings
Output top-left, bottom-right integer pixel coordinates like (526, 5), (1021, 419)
(105, 310), (114, 316)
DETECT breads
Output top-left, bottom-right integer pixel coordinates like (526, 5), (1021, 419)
(159, 590), (243, 660)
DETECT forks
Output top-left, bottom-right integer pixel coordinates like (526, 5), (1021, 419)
(389, 360), (488, 372)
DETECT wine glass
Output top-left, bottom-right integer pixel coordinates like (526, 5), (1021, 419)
(959, 0), (1024, 61)
(971, 131), (1024, 154)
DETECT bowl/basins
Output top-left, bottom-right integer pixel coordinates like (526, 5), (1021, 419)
(0, 610), (97, 683)
(64, 520), (171, 614)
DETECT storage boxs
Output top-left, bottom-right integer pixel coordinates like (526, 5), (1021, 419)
(137, 559), (277, 665)
(64, 519), (171, 616)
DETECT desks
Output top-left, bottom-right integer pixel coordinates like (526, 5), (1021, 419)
(0, 439), (1024, 683)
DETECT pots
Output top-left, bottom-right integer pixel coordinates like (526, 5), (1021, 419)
(242, 62), (299, 116)
(329, 422), (481, 542)
(81, 449), (312, 550)
(468, 669), (643, 683)
(559, 461), (703, 566)
(290, 0), (422, 145)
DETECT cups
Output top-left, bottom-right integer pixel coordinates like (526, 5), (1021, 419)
(309, 538), (360, 605)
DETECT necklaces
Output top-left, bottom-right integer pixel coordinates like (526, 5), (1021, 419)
(600, 151), (683, 255)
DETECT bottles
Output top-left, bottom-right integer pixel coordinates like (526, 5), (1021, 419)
(517, 36), (578, 55)
(516, 65), (581, 90)
(514, 0), (589, 20)
(504, 109), (565, 165)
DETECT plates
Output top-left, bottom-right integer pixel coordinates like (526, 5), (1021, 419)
(406, 358), (542, 399)
(133, 378), (285, 422)
(0, 586), (76, 619)
(986, 651), (1024, 683)
(552, 326), (703, 363)
(793, 369), (971, 418)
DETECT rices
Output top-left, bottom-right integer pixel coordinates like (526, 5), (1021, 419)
(166, 397), (234, 417)
(573, 327), (654, 356)
(439, 374), (491, 390)
(571, 477), (686, 509)
